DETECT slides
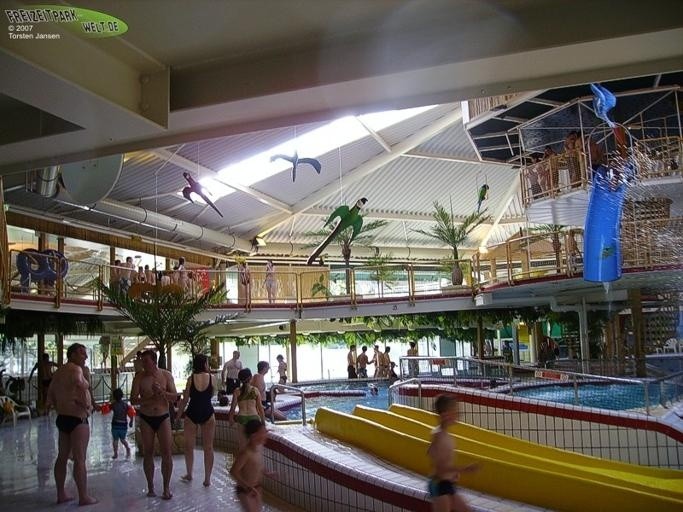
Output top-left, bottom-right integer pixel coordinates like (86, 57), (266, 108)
(583, 162), (631, 283)
(315, 402), (683, 512)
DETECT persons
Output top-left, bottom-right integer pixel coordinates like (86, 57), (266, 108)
(240, 262), (250, 302)
(526, 130), (623, 198)
(45, 343), (99, 505)
(427, 393), (478, 512)
(484, 341), (491, 356)
(347, 343), (419, 395)
(566, 229), (582, 271)
(115, 257), (197, 301)
(536, 340), (556, 368)
(27, 354), (60, 416)
(92, 388), (134, 458)
(262, 260), (276, 304)
(131, 349), (288, 512)
(503, 341), (513, 363)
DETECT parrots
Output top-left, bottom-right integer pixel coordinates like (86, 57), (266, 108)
(269, 151), (320, 182)
(307, 197), (367, 266)
(476, 184), (489, 214)
(181, 172), (223, 217)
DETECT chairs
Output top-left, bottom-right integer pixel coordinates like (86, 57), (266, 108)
(0, 396), (32, 428)
(663, 338), (677, 353)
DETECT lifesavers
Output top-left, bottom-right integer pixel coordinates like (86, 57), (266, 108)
(17, 248), (46, 278)
(40, 250), (67, 280)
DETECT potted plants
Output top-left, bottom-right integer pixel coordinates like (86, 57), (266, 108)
(296, 197), (389, 302)
(410, 200), (494, 295)
(92, 278), (230, 455)
(527, 224), (576, 276)
